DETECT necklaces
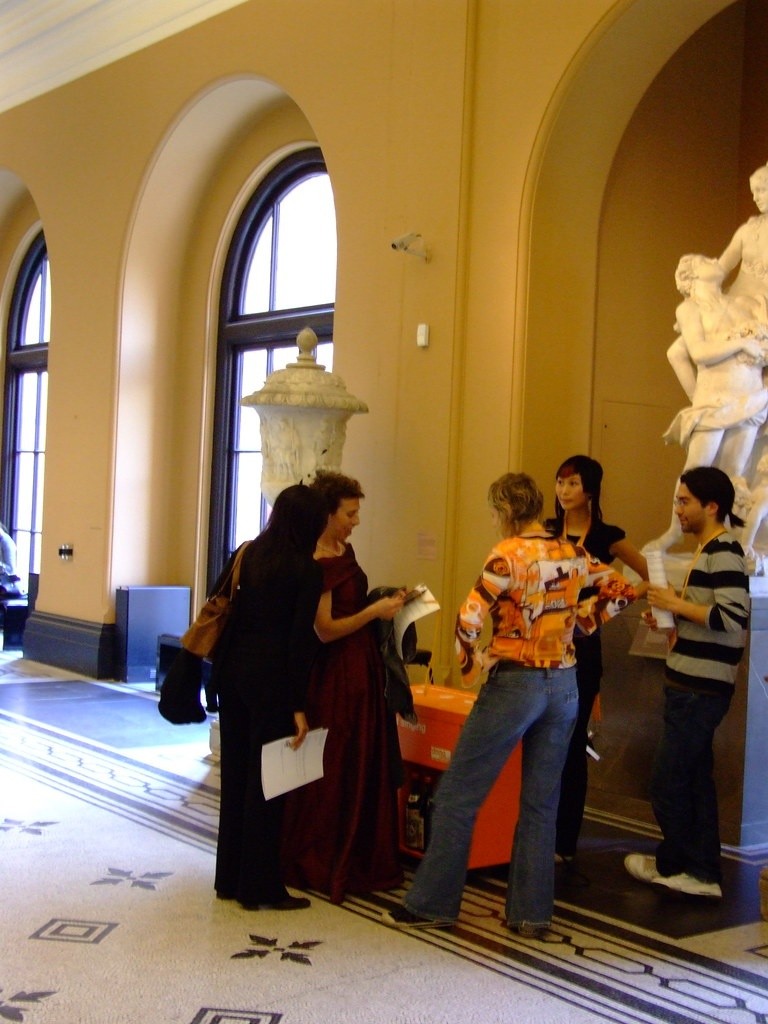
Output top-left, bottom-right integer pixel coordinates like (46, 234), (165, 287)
(315, 538), (342, 556)
(518, 528), (547, 533)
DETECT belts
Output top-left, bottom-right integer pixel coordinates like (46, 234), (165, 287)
(490, 660), (542, 673)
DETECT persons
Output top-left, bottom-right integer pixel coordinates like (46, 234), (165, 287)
(540, 455), (648, 862)
(635, 160), (768, 573)
(0, 522), (17, 574)
(383, 471), (637, 938)
(622, 466), (751, 898)
(211, 470), (419, 912)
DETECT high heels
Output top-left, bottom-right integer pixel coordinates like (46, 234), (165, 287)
(239, 883), (310, 909)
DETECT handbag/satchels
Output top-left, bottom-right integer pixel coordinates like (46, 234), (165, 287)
(180, 540), (253, 656)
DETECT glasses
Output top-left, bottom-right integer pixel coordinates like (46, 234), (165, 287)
(674, 498), (700, 506)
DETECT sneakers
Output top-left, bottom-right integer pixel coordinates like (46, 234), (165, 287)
(625, 852), (660, 883)
(651, 872), (723, 898)
(383, 905), (455, 928)
(519, 920), (540, 938)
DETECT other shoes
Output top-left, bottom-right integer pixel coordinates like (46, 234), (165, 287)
(555, 852), (574, 865)
